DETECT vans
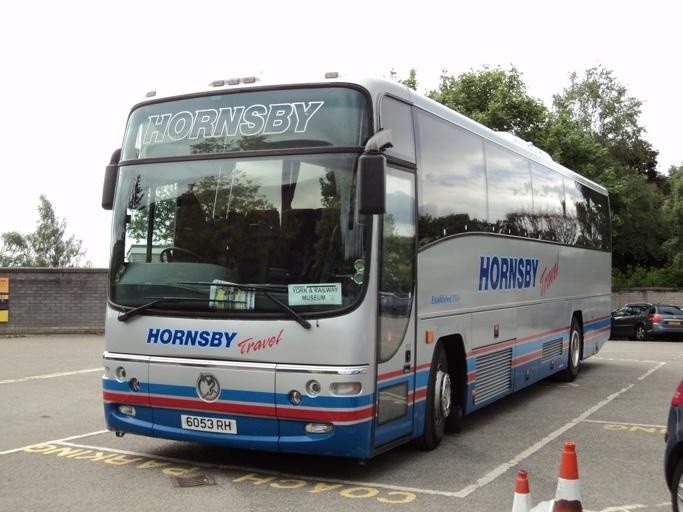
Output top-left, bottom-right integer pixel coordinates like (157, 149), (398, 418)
(611, 302), (653, 341)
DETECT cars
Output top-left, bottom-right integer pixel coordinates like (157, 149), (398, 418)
(643, 305), (682, 341)
(662, 379), (682, 511)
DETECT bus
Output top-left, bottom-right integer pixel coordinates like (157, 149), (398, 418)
(100, 72), (613, 462)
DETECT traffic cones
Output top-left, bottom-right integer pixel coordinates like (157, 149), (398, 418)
(511, 469), (531, 512)
(552, 442), (585, 511)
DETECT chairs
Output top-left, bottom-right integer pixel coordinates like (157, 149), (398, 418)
(244, 207), (339, 276)
